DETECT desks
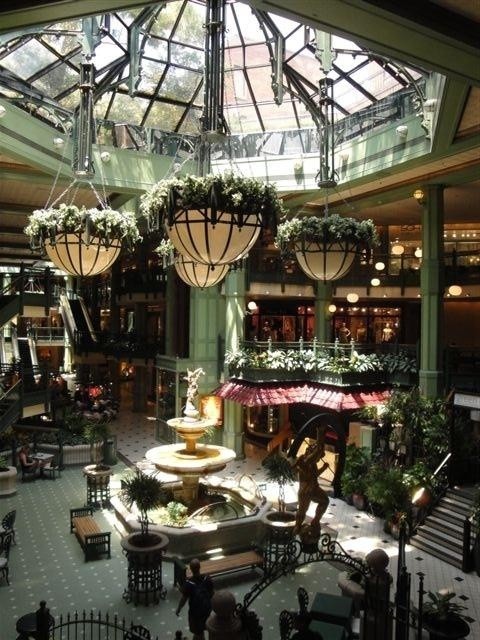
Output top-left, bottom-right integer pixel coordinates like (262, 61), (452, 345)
(306, 569), (365, 640)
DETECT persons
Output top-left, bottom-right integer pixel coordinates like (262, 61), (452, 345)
(18, 444), (51, 473)
(175, 558), (214, 640)
(261, 321), (272, 342)
(291, 440), (330, 536)
(337, 322), (351, 343)
(354, 322), (366, 343)
(380, 322), (395, 342)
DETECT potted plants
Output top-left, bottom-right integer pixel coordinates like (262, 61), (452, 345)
(0, 456), (21, 498)
(142, 176), (286, 264)
(259, 448), (299, 546)
(155, 239), (248, 288)
(83, 421), (112, 488)
(120, 467), (170, 571)
(342, 444), (401, 517)
(24, 176), (139, 278)
(276, 176), (382, 281)
(417, 591), (472, 640)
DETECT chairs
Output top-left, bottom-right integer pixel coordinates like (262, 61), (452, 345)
(17, 444), (63, 483)
(0, 509), (20, 586)
(278, 587), (311, 640)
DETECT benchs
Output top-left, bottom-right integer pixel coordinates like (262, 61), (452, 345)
(173, 544), (262, 589)
(70, 507), (110, 561)
(270, 501), (338, 544)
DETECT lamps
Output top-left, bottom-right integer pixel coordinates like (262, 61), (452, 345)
(412, 189), (427, 208)
(245, 301), (257, 317)
